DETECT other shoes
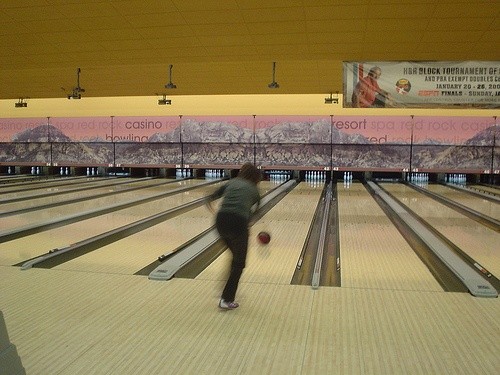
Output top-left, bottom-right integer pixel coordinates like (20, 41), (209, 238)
(219, 299), (239, 309)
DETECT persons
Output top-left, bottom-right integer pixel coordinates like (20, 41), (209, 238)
(351, 66), (392, 108)
(203, 161), (260, 307)
